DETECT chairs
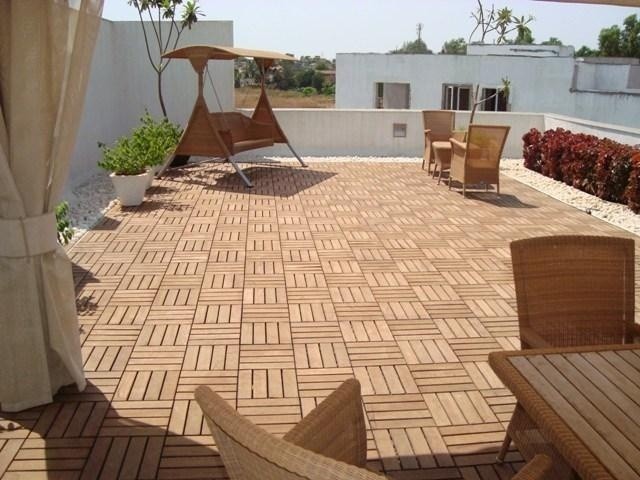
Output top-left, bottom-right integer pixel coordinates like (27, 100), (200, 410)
(498, 235), (640, 464)
(421, 106), (511, 198)
(192, 379), (554, 478)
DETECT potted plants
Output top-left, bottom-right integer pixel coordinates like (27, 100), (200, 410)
(95, 0), (208, 207)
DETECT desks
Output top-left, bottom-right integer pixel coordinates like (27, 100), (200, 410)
(487, 342), (640, 480)
